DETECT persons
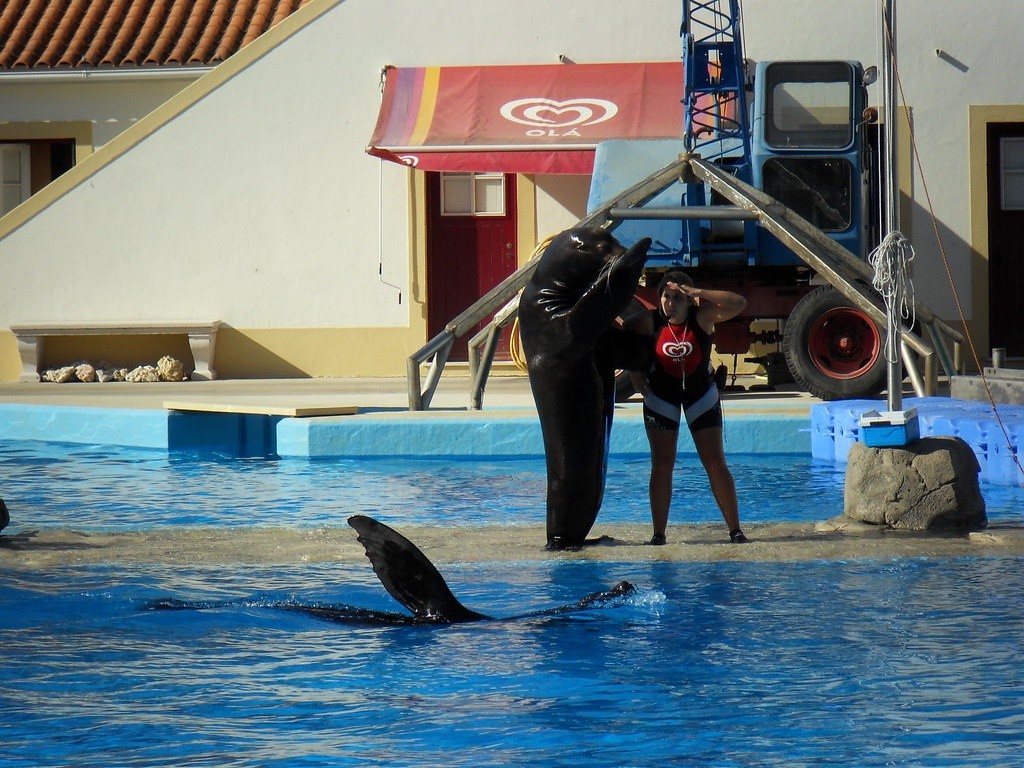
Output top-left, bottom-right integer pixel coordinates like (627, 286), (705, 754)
(621, 271), (749, 544)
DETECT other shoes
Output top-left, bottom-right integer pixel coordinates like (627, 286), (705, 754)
(729, 528), (750, 544)
(644, 533), (667, 547)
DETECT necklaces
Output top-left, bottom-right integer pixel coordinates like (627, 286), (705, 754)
(667, 318), (688, 389)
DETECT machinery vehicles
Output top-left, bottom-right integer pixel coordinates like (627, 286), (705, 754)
(584, 0), (891, 400)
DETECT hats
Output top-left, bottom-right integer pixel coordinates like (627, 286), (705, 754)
(658, 270), (694, 298)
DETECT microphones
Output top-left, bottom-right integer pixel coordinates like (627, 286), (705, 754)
(658, 305), (670, 320)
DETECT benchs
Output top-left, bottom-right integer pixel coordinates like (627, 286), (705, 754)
(10, 319), (222, 383)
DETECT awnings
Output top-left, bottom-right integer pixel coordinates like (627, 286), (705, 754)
(366, 63), (740, 173)
